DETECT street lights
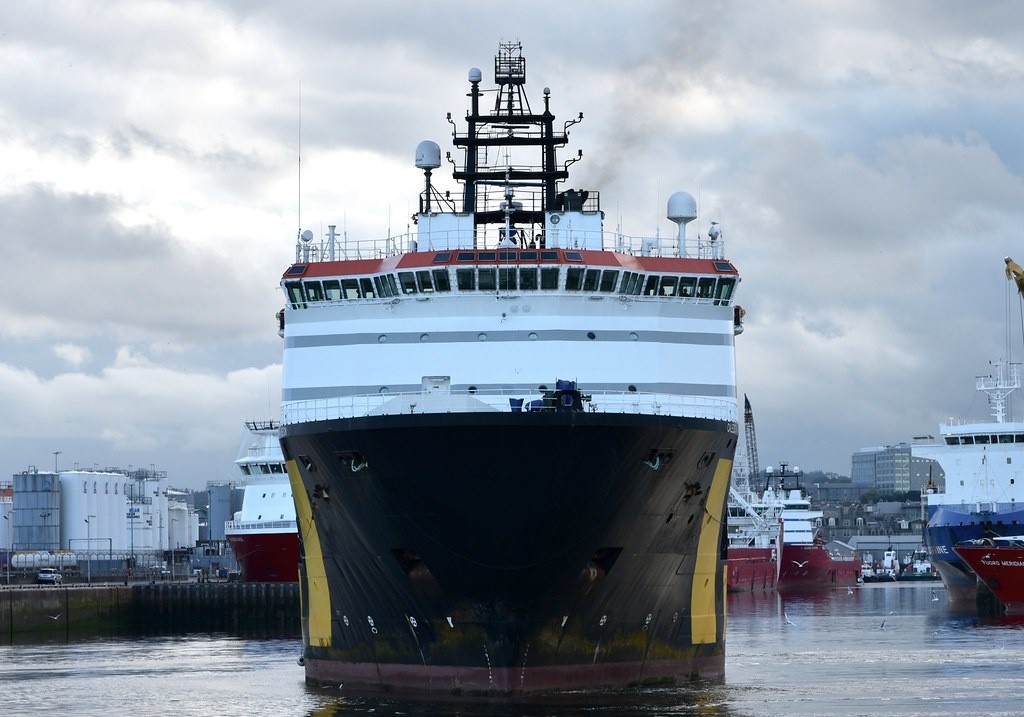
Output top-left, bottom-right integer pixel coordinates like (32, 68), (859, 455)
(128, 483), (136, 567)
(4, 511), (16, 584)
(171, 518), (180, 580)
(84, 515), (97, 581)
(208, 489), (212, 574)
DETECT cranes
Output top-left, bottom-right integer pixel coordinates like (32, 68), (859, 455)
(743, 392), (761, 495)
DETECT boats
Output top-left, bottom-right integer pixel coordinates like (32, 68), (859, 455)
(275, 37), (747, 707)
(860, 545), (939, 582)
(222, 420), (303, 584)
(910, 255), (1024, 614)
(727, 462), (861, 595)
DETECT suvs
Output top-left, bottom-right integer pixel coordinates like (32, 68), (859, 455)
(38, 567), (63, 585)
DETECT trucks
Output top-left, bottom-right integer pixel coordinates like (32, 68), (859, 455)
(191, 546), (220, 570)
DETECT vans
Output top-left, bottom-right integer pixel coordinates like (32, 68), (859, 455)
(151, 565), (171, 575)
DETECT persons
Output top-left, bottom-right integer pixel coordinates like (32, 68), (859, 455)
(127, 568), (132, 576)
(887, 545), (893, 551)
(539, 385), (555, 413)
(891, 559), (895, 569)
(906, 565), (912, 572)
(931, 565), (936, 576)
(872, 559), (882, 574)
(813, 529), (825, 544)
